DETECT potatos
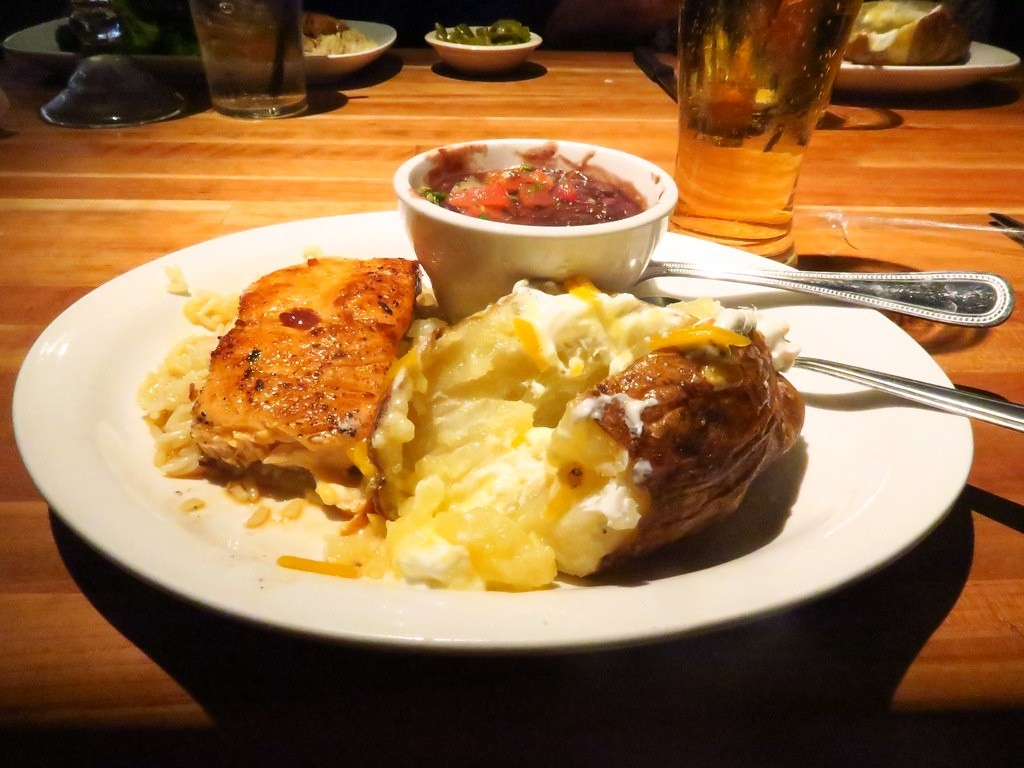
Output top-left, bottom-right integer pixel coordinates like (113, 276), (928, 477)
(842, 0), (972, 66)
(372, 281), (807, 587)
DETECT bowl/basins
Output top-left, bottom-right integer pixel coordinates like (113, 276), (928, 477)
(425, 26), (543, 72)
(395, 138), (675, 327)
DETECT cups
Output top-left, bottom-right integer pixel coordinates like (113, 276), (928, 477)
(188, 1), (308, 119)
(666, 0), (860, 266)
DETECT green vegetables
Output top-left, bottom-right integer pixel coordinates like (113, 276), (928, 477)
(422, 162), (544, 222)
(104, 0), (204, 59)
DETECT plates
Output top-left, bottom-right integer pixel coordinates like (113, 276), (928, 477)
(837, 40), (1020, 90)
(10, 210), (976, 648)
(4, 17), (397, 84)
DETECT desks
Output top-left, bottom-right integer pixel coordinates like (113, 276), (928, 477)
(0, 51), (1024, 753)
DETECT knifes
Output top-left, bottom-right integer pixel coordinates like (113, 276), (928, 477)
(633, 48), (677, 102)
(987, 213), (1024, 241)
